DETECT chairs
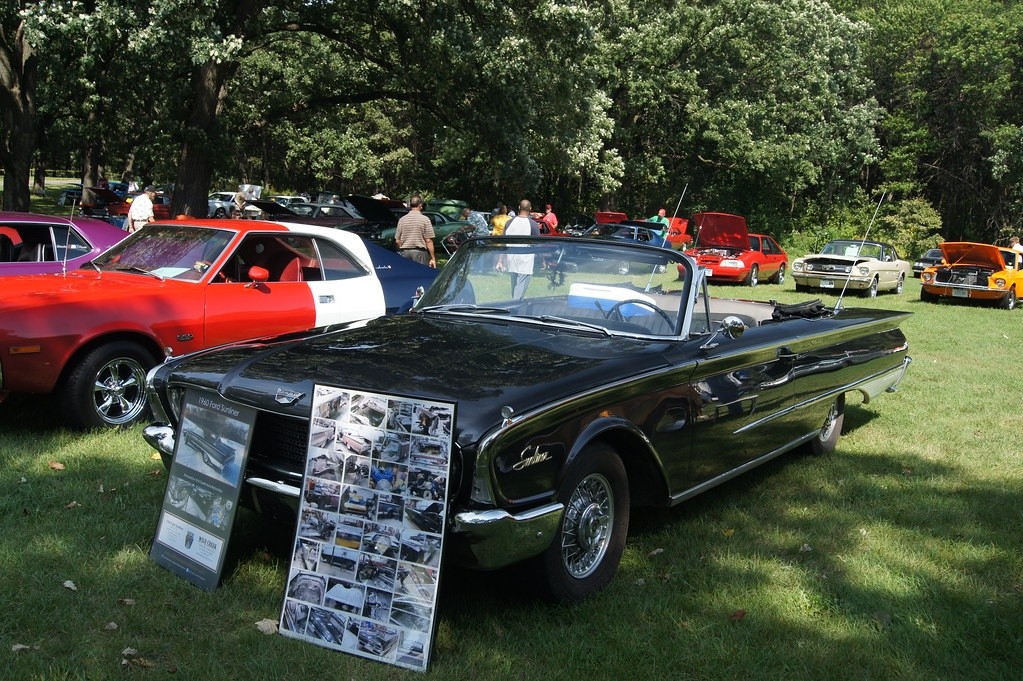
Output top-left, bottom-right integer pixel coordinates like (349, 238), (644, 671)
(18, 235), (47, 261)
(240, 239), (283, 282)
(516, 299), (757, 335)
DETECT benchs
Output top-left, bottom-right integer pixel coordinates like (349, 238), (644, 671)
(302, 267), (348, 280)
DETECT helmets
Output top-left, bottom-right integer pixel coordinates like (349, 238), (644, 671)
(145, 186), (159, 193)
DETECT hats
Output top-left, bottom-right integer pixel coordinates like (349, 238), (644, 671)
(545, 205), (551, 209)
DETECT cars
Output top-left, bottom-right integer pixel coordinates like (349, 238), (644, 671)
(912, 241), (1023, 308)
(524, 211), (693, 275)
(343, 497), (369, 514)
(791, 238), (909, 297)
(184, 430), (236, 471)
(338, 431), (373, 453)
(210, 183), (474, 254)
(677, 212), (787, 285)
(0, 211), (477, 428)
(60, 181), (172, 217)
(143, 236), (915, 596)
(358, 627), (395, 657)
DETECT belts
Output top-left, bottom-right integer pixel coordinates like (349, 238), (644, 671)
(134, 219), (147, 222)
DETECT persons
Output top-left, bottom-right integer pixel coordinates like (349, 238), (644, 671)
(129, 185), (155, 233)
(497, 199), (547, 300)
(1009, 237), (1023, 269)
(538, 205), (558, 234)
(331, 195), (343, 205)
(461, 200), (515, 273)
(646, 209), (669, 240)
(395, 195), (436, 269)
(234, 188), (253, 218)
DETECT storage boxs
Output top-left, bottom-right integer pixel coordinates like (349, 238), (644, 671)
(568, 282), (655, 318)
